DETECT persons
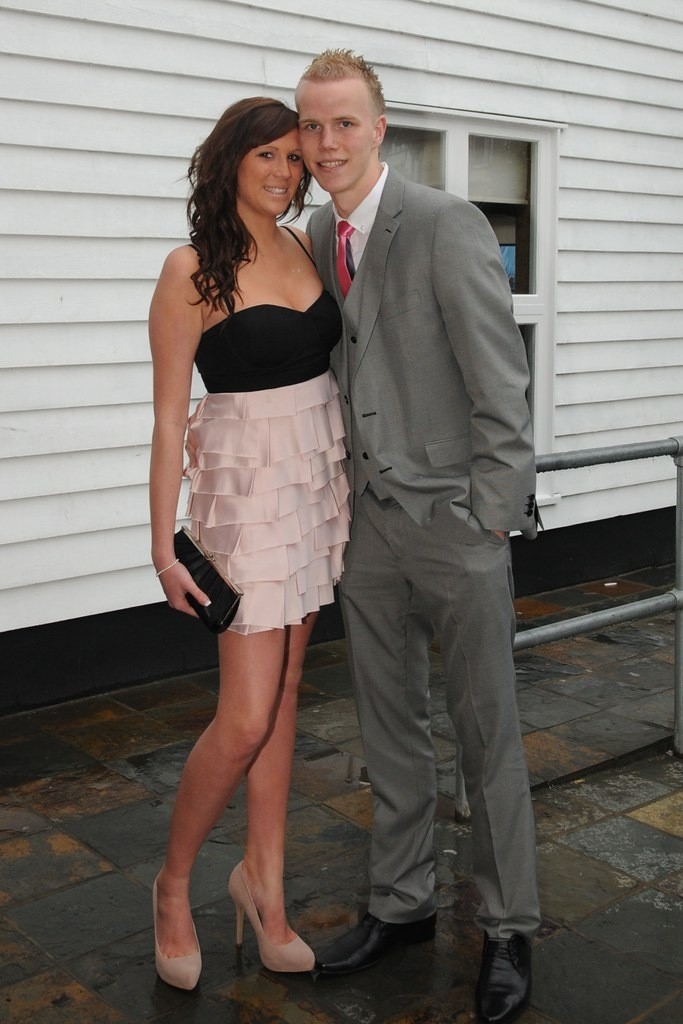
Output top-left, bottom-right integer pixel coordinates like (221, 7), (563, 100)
(148, 96), (349, 988)
(295, 48), (543, 1024)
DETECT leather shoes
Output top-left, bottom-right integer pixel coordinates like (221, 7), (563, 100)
(478, 929), (531, 1024)
(316, 909), (438, 974)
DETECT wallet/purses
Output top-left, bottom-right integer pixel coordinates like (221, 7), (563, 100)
(172, 524), (244, 634)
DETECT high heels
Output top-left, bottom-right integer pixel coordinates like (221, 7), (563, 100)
(152, 870), (202, 991)
(229, 859), (316, 973)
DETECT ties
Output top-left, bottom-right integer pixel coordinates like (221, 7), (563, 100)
(335, 219), (358, 295)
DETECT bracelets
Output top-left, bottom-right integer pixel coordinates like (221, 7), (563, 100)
(156, 559), (179, 577)
